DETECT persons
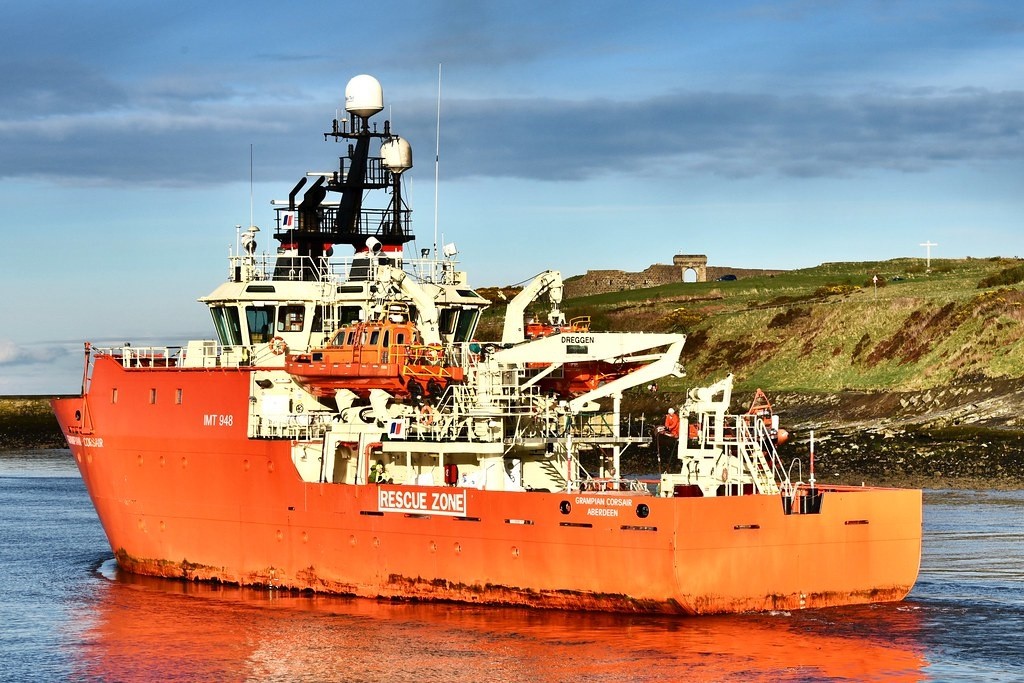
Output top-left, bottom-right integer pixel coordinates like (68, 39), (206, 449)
(369, 463), (395, 485)
(665, 407), (698, 439)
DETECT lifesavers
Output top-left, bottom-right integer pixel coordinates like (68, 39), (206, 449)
(419, 405), (434, 426)
(425, 343), (442, 361)
(408, 341), (425, 360)
(269, 336), (286, 355)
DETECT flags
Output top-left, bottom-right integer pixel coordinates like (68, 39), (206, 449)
(280, 210), (295, 229)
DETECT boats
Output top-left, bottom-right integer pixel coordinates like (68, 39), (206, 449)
(49, 75), (926, 618)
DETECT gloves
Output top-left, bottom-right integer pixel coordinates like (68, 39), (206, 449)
(664, 427), (669, 431)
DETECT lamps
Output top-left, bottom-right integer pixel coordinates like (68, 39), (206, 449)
(240, 231), (257, 253)
(365, 236), (382, 256)
(443, 243), (457, 258)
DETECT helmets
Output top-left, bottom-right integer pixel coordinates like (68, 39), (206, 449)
(668, 408), (675, 413)
